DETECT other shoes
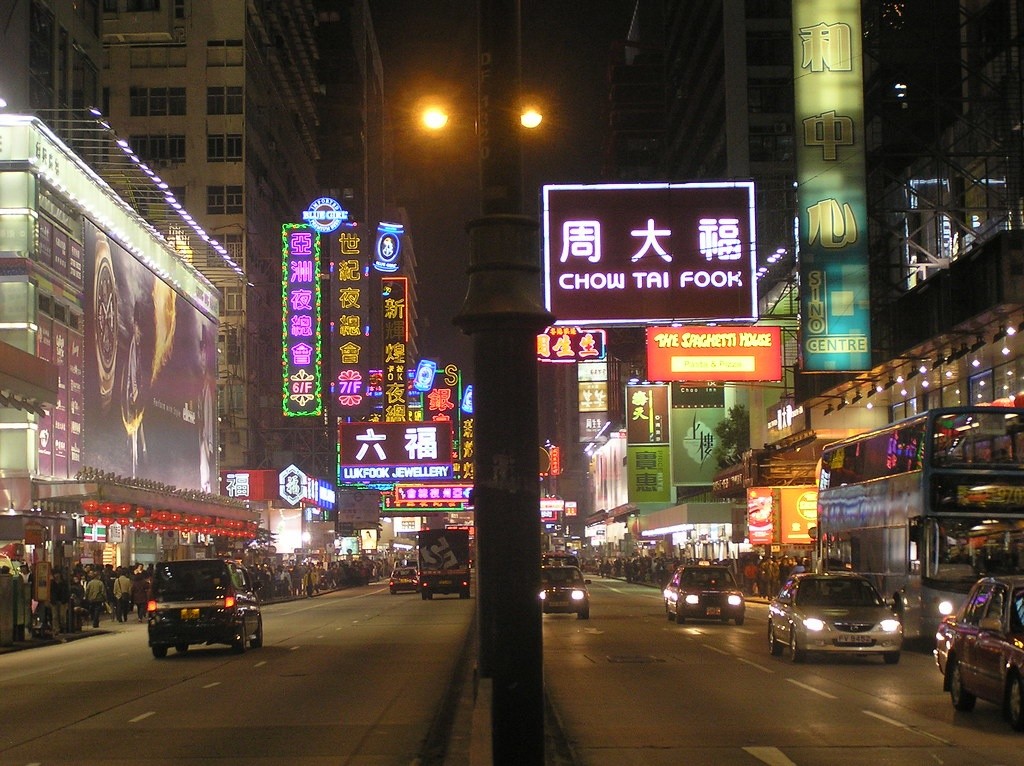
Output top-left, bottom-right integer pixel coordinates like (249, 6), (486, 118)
(93, 624), (99, 628)
(139, 619), (142, 624)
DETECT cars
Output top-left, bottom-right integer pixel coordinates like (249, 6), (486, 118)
(934, 578), (1024, 734)
(768, 572), (904, 664)
(662, 565), (746, 624)
(389, 568), (418, 594)
(539, 566), (591, 620)
(396, 558), (419, 571)
(542, 554), (577, 568)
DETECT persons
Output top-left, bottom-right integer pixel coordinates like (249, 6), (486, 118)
(70, 563), (162, 626)
(86, 571), (107, 628)
(51, 572), (69, 632)
(130, 573), (150, 624)
(581, 552), (811, 602)
(249, 557), (377, 601)
(114, 569), (131, 622)
(1, 566), (10, 574)
(17, 561), (32, 583)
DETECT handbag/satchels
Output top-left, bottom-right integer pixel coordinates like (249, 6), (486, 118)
(122, 592), (129, 602)
(105, 602), (112, 614)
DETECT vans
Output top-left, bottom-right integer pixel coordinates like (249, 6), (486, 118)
(147, 559), (263, 657)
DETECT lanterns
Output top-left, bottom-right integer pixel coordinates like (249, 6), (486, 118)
(99, 502), (115, 515)
(84, 514), (98, 525)
(133, 520), (145, 530)
(145, 520), (157, 532)
(159, 511), (257, 539)
(117, 516), (129, 527)
(115, 502), (131, 516)
(131, 506), (146, 519)
(101, 516), (115, 526)
(84, 499), (99, 512)
(151, 510), (159, 519)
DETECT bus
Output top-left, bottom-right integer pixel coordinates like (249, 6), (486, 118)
(815, 405), (1024, 646)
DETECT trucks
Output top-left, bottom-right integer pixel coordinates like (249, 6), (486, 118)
(418, 529), (471, 600)
